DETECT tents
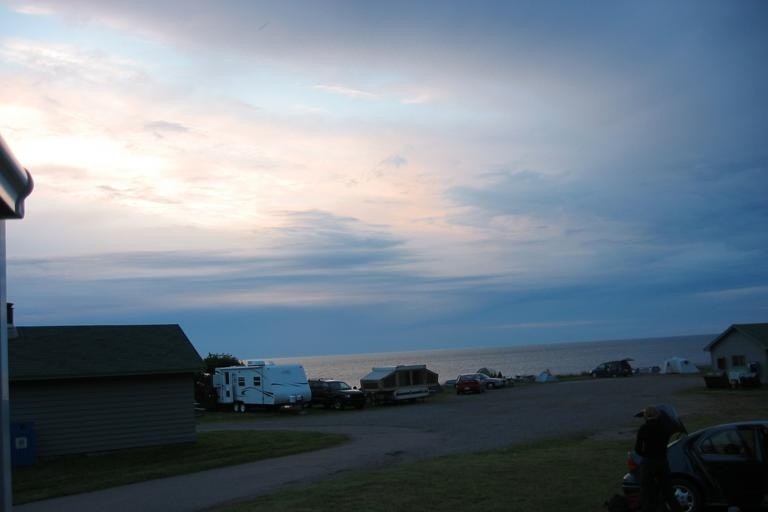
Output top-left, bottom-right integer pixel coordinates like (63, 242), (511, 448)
(534, 368), (561, 383)
(658, 355), (701, 375)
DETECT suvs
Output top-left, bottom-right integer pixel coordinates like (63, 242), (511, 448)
(307, 377), (367, 411)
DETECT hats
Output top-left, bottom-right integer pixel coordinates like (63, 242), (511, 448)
(643, 405), (661, 420)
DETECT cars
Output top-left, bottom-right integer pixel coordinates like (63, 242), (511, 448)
(622, 403), (768, 512)
(454, 373), (507, 395)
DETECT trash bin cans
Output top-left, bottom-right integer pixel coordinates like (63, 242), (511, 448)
(9, 420), (37, 468)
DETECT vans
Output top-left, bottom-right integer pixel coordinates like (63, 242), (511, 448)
(589, 358), (634, 378)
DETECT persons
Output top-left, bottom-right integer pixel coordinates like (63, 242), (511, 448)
(633, 404), (684, 512)
